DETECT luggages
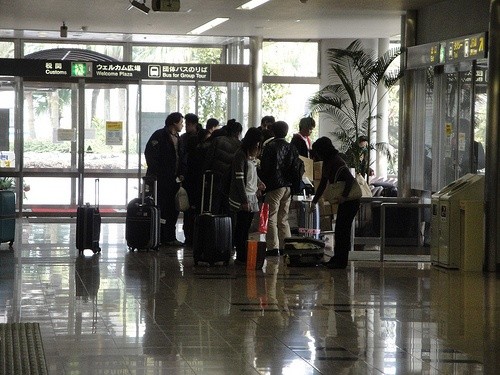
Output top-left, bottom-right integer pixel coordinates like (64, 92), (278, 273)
(192, 171), (232, 266)
(76, 179), (101, 255)
(126, 176), (161, 252)
(0, 191), (16, 246)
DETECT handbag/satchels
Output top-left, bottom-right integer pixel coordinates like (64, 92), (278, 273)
(324, 166), (362, 204)
(355, 173), (373, 197)
(176, 187), (190, 212)
(249, 202), (268, 233)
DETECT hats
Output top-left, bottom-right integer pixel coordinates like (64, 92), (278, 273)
(360, 136), (369, 141)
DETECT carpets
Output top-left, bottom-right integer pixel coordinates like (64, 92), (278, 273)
(31, 208), (117, 212)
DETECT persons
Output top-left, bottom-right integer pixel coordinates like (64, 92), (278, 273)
(229, 127), (266, 261)
(144, 112), (184, 248)
(452, 119), (485, 175)
(257, 121), (293, 256)
(290, 117), (315, 158)
(179, 113), (275, 256)
(309, 137), (362, 269)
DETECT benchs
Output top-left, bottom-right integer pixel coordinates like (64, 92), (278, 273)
(0, 322), (50, 375)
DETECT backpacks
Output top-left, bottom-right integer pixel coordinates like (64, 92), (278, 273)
(273, 140), (305, 184)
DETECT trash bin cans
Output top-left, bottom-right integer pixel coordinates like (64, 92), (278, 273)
(428, 172), (484, 269)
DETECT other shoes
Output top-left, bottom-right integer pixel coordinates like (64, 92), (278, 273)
(165, 241), (184, 246)
(320, 261), (334, 266)
(279, 249), (285, 255)
(327, 264), (347, 269)
(266, 249), (278, 256)
